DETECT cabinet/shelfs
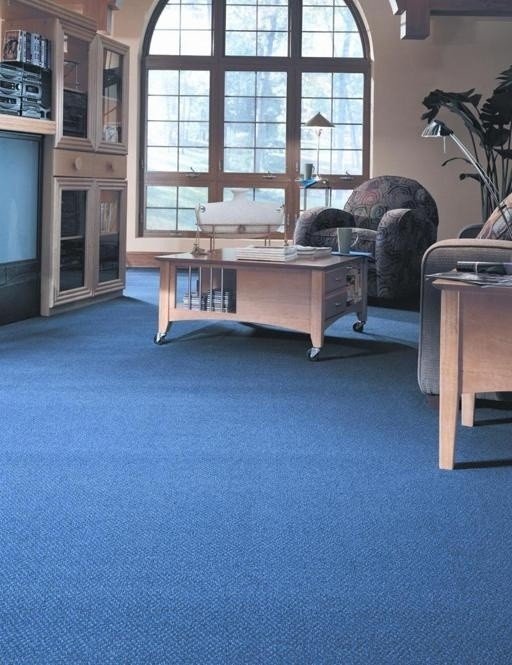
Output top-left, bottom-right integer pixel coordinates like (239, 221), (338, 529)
(49, 148), (127, 315)
(3, 0), (57, 135)
(58, 18), (130, 153)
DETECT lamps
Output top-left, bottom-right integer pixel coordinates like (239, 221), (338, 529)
(422, 118), (511, 254)
(302, 112), (337, 181)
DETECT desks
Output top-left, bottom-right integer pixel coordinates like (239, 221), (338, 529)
(427, 269), (512, 472)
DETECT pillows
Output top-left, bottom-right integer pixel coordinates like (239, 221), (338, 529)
(473, 193), (512, 240)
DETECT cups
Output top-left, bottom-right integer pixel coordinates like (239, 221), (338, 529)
(304, 163), (315, 180)
(336, 227), (359, 254)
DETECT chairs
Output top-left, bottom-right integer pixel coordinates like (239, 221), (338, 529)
(417, 192), (512, 396)
(292, 175), (440, 305)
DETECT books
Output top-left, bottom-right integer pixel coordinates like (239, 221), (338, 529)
(236, 244), (332, 262)
(2, 30), (51, 69)
(183, 288), (233, 312)
